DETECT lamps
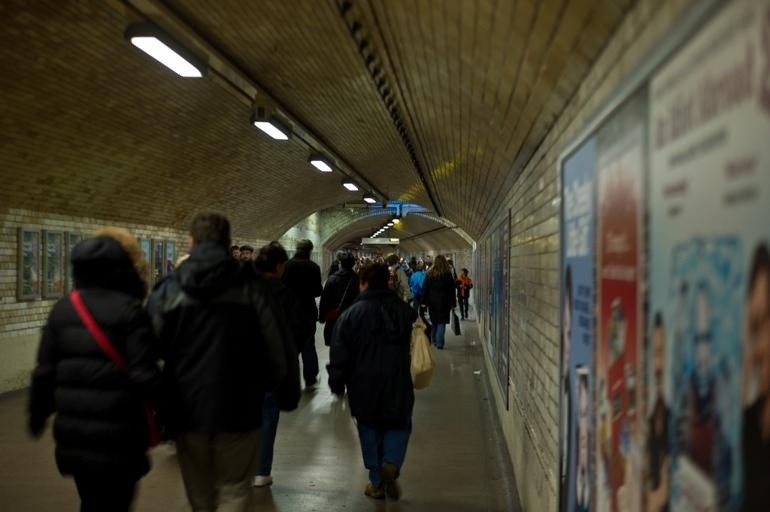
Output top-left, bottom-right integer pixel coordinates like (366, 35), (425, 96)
(125, 21), (206, 78)
(309, 155), (334, 173)
(253, 119), (291, 140)
(342, 179), (360, 192)
(363, 194), (376, 203)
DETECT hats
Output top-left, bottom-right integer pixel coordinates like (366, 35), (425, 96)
(296, 239), (313, 250)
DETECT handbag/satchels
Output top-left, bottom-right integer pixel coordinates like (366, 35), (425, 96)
(409, 316), (436, 390)
(451, 309), (461, 336)
(325, 309), (339, 323)
(141, 399), (161, 448)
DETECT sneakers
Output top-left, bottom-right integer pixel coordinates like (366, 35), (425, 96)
(255, 476), (272, 487)
(364, 463), (399, 499)
(306, 375), (320, 393)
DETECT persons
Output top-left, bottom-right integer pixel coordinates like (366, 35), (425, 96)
(224, 242), (304, 487)
(325, 263), (432, 501)
(559, 243), (770, 512)
(229, 239), (473, 399)
(144, 210), (290, 512)
(26, 227), (166, 512)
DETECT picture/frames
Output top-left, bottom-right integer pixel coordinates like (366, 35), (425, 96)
(553, 0), (770, 512)
(17, 228), (177, 302)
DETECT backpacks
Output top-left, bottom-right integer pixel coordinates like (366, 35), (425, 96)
(387, 269), (400, 290)
